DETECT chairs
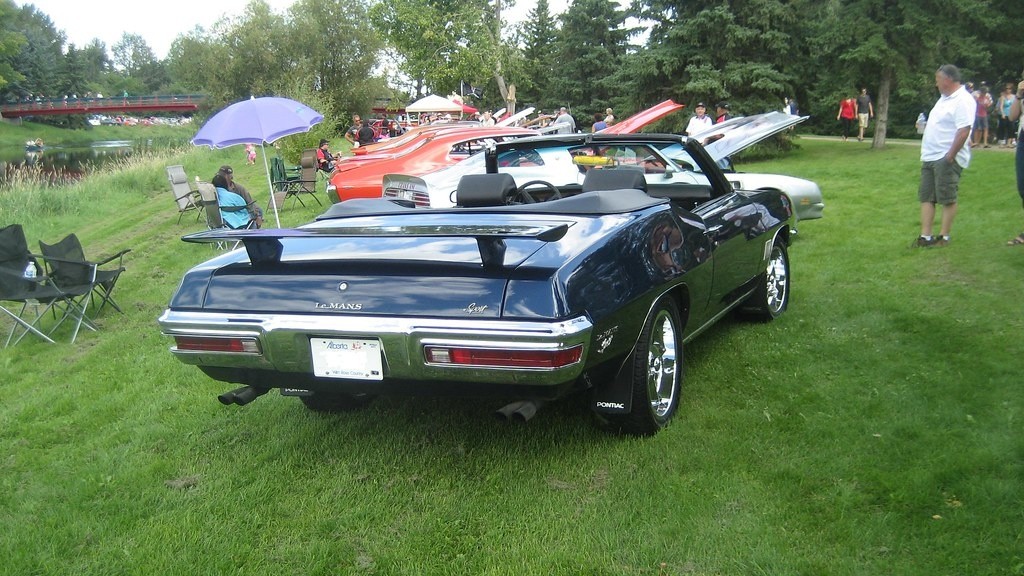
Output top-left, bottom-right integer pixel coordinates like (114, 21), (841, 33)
(456, 174), (519, 207)
(582, 169), (648, 195)
(0, 224), (131, 348)
(166, 165), (260, 251)
(264, 148), (340, 214)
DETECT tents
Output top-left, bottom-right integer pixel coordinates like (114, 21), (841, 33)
(406, 92), (464, 127)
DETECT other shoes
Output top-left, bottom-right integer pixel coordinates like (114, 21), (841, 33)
(911, 234), (931, 247)
(931, 234), (950, 246)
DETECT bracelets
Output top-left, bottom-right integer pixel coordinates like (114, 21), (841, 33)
(1016, 94), (1022, 100)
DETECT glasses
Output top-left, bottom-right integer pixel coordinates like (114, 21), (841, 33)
(221, 167), (232, 174)
(355, 119), (361, 123)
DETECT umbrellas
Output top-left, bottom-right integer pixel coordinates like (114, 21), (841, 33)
(187, 94), (324, 227)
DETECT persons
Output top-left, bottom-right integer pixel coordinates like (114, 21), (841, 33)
(994, 92), (1004, 144)
(912, 63), (978, 250)
(245, 143), (257, 165)
(345, 114), (379, 155)
(836, 97), (858, 139)
(965, 81), (993, 148)
(317, 139), (348, 173)
(210, 175), (258, 230)
(354, 119), (378, 145)
(686, 102), (712, 138)
(212, 165), (263, 229)
(15, 89), (130, 109)
(1006, 70), (1024, 248)
(998, 82), (1016, 146)
(467, 106), (613, 134)
(704, 101), (731, 170)
(783, 97), (800, 131)
(855, 87), (873, 141)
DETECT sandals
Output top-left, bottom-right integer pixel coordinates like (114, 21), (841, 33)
(1007, 233), (1024, 245)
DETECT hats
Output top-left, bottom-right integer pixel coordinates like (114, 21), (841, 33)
(715, 101), (732, 110)
(475, 111), (480, 115)
(319, 138), (330, 146)
(220, 165), (233, 180)
(696, 102), (707, 108)
(437, 113), (442, 117)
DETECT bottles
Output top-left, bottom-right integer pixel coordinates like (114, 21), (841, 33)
(917, 113), (926, 134)
(25, 262), (37, 278)
(195, 176), (200, 185)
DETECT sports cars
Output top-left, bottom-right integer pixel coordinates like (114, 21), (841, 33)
(326, 97), (686, 206)
(381, 110), (827, 220)
(157, 132), (799, 439)
